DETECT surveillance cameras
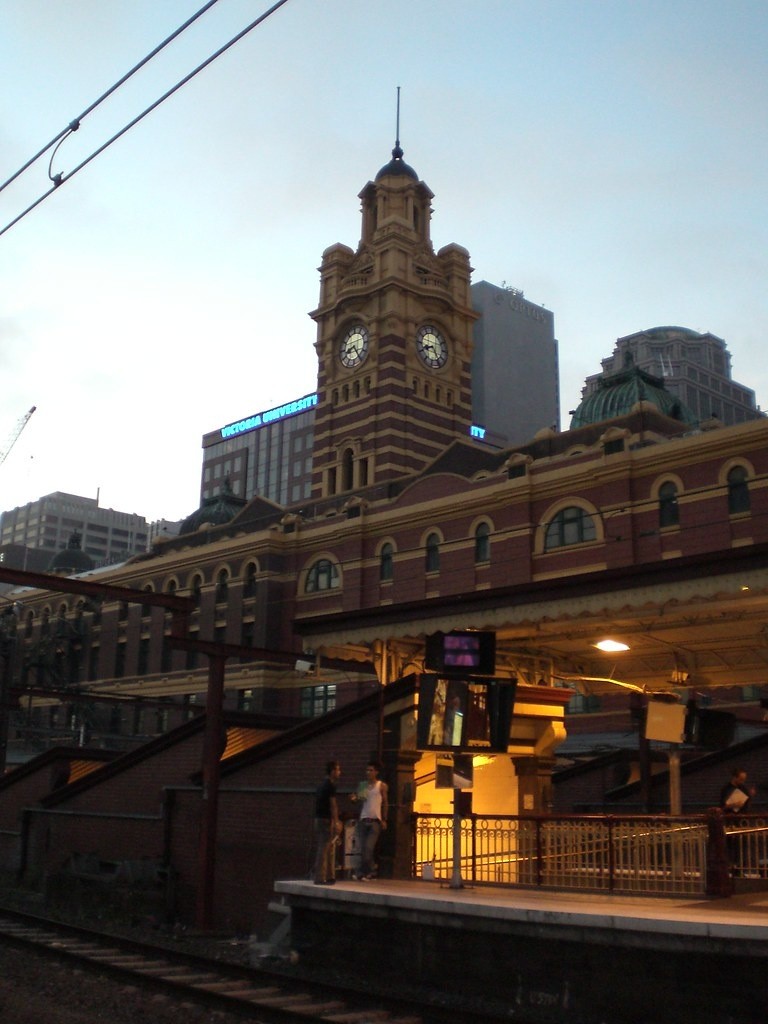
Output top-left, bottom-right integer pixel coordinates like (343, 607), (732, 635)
(672, 672), (692, 683)
(294, 659), (318, 674)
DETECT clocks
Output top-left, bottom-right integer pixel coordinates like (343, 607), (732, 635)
(414, 321), (453, 375)
(334, 320), (369, 374)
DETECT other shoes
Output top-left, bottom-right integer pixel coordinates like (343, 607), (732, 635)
(362, 877), (369, 882)
(326, 879), (336, 885)
(314, 881), (326, 885)
(352, 874), (357, 880)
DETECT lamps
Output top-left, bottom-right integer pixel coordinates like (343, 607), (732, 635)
(587, 635), (631, 652)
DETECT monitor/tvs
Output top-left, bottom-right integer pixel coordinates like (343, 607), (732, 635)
(424, 631), (496, 675)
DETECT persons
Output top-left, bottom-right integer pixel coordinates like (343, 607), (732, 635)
(720, 767), (756, 878)
(313, 761), (344, 884)
(348, 761), (388, 881)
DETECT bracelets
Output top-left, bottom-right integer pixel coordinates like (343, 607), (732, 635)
(382, 816), (387, 821)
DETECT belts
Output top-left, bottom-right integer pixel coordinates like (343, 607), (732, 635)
(362, 818), (379, 822)
